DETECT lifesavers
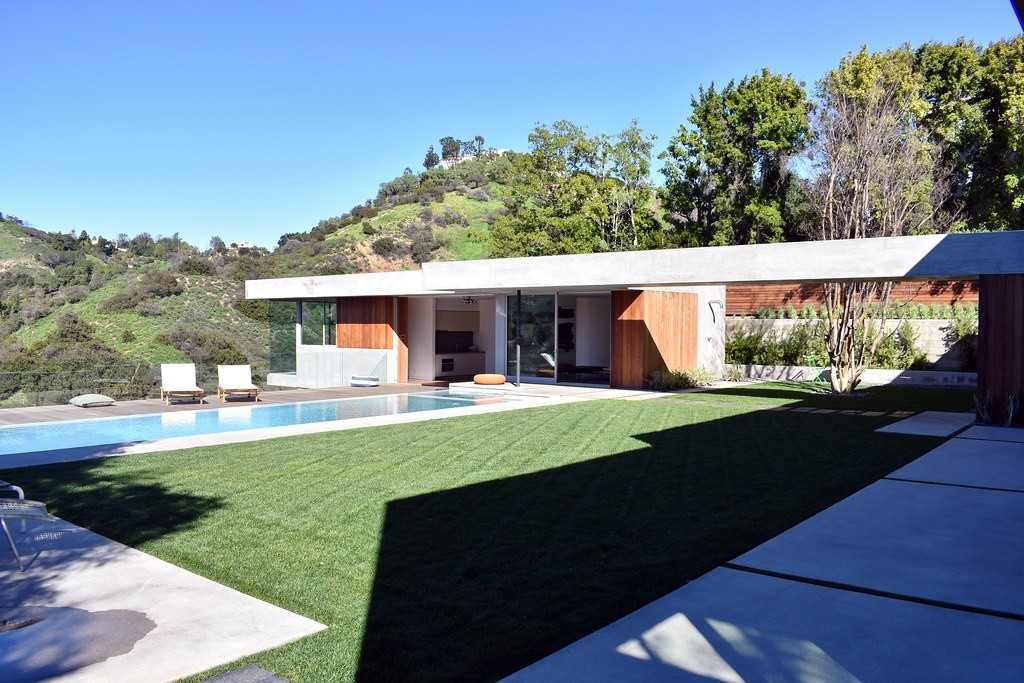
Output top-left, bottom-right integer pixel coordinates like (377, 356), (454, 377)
(473, 373), (507, 385)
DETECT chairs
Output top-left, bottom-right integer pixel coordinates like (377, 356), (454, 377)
(216, 364), (259, 404)
(0, 485), (56, 572)
(160, 363), (204, 406)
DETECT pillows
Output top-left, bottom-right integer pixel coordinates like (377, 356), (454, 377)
(68, 393), (116, 408)
